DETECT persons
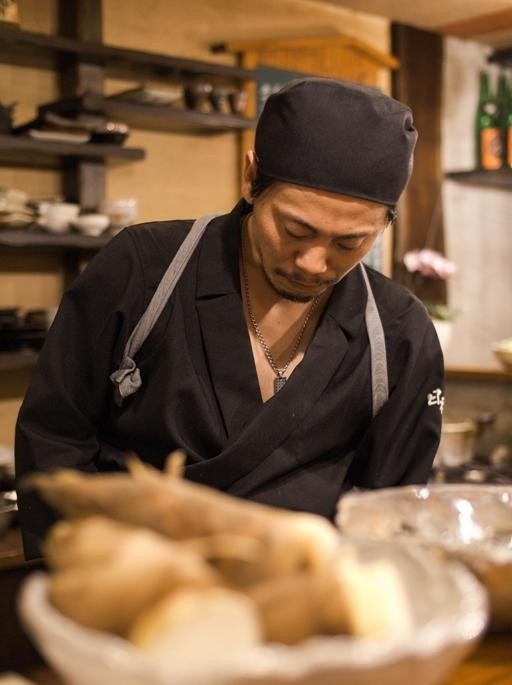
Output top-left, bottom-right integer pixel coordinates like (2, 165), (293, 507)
(14, 75), (446, 565)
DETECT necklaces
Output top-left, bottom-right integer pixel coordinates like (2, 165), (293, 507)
(244, 247), (325, 396)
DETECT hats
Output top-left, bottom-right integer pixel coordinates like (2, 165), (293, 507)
(254, 78), (418, 207)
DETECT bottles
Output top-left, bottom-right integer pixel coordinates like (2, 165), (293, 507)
(474, 71), (512, 170)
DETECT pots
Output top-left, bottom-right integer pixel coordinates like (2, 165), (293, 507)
(433, 418), (479, 474)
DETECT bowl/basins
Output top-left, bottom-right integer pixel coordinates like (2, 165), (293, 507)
(79, 213), (108, 238)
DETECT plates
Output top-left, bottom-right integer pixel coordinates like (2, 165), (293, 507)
(344, 483), (511, 610)
(21, 540), (467, 680)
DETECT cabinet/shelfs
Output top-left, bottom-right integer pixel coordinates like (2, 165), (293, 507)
(0, 0), (260, 374)
